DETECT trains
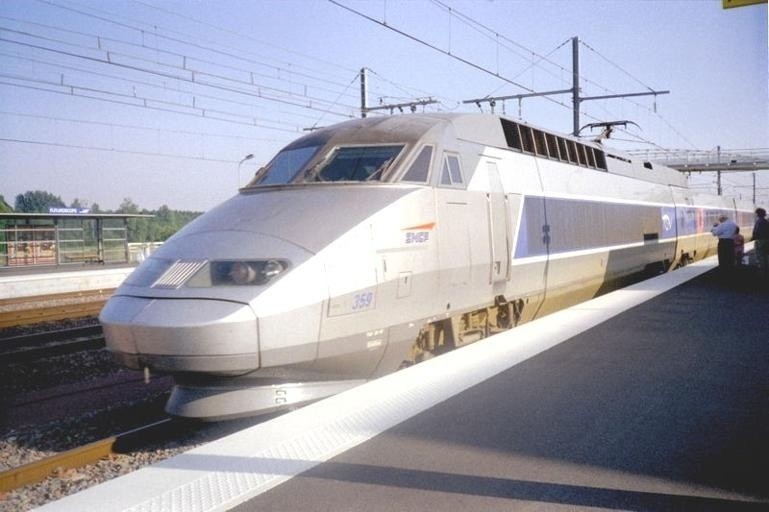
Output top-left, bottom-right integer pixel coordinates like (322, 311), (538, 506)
(98, 111), (769, 428)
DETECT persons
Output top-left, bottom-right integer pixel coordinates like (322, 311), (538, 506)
(710, 215), (736, 268)
(735, 226), (744, 255)
(752, 207), (769, 274)
